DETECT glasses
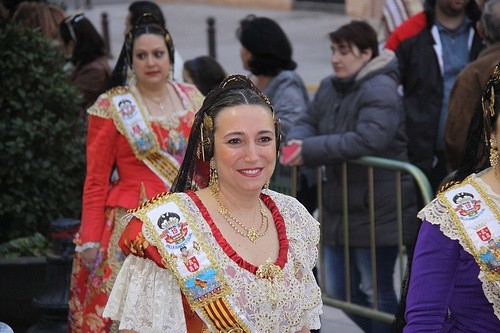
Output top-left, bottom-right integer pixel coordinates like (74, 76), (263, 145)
(65, 12), (84, 43)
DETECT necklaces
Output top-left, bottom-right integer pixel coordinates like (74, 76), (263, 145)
(212, 192), (270, 244)
(141, 85), (178, 126)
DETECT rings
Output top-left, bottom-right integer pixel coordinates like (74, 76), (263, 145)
(85, 263), (89, 266)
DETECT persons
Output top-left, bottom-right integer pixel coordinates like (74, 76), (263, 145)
(0, 0), (500, 333)
(101, 74), (323, 333)
